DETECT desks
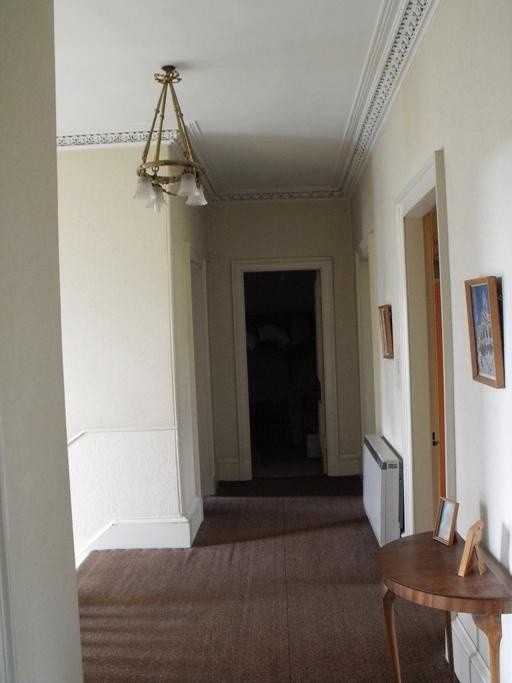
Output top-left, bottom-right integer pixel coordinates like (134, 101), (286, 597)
(373, 530), (511, 683)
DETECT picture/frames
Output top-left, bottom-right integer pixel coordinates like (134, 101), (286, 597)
(377, 304), (393, 359)
(432, 496), (484, 577)
(463, 276), (505, 389)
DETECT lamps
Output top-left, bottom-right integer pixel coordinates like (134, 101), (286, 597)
(129, 63), (213, 217)
